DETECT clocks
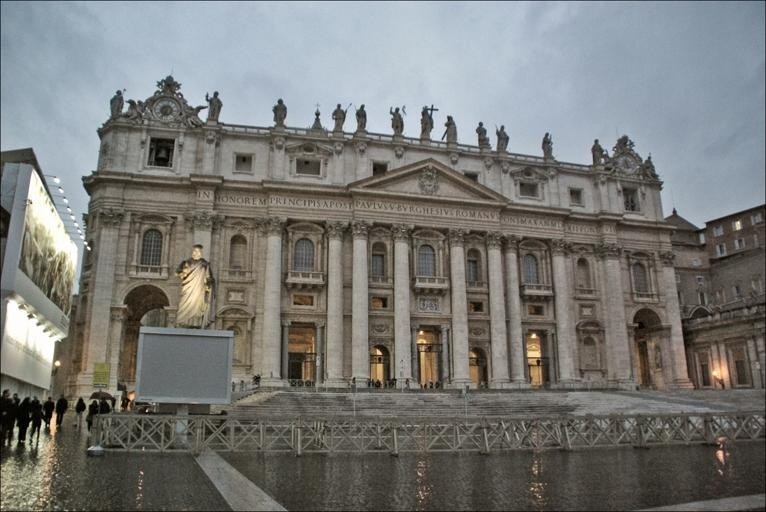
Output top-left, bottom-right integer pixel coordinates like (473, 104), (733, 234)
(616, 152), (637, 174)
(151, 96), (182, 123)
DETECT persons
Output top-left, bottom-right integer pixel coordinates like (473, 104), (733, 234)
(390, 105), (404, 135)
(591, 140), (610, 167)
(445, 116), (457, 143)
(109, 89), (125, 118)
(121, 395), (130, 410)
(429, 379), (434, 388)
(435, 380), (440, 388)
(369, 377), (381, 388)
(205, 91), (223, 122)
(475, 121), (492, 146)
(645, 156), (655, 171)
(232, 380), (235, 391)
(494, 125), (509, 151)
(332, 103), (347, 132)
(272, 98), (287, 126)
(420, 107), (434, 138)
(239, 380), (245, 391)
(355, 104), (367, 130)
(424, 382), (427, 390)
(175, 244), (215, 329)
(1, 388), (115, 445)
(541, 132), (555, 160)
